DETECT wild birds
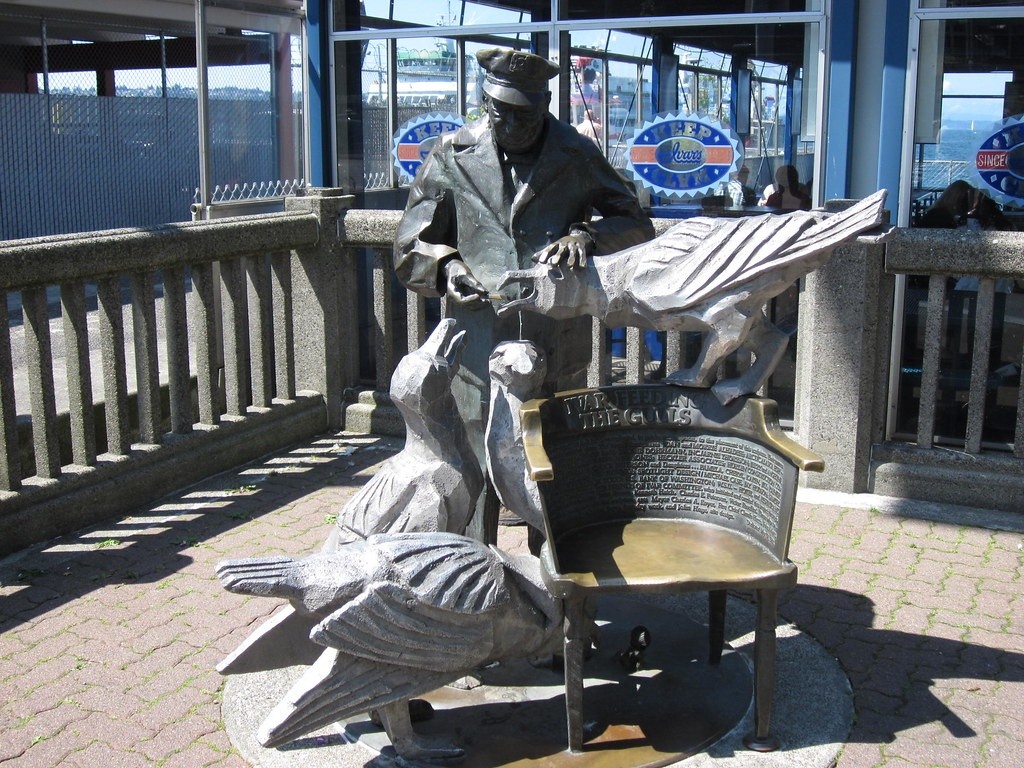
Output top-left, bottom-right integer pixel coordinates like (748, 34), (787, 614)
(214, 319), (468, 671)
(209, 528), (584, 761)
(497, 188), (895, 407)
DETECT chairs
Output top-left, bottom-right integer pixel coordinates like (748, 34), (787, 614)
(520, 381), (825, 754)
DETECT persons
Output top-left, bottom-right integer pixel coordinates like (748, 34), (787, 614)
(913, 181), (974, 289)
(577, 109), (601, 139)
(701, 165), (757, 209)
(392, 49), (655, 689)
(955, 187), (1018, 295)
(767, 165), (811, 210)
